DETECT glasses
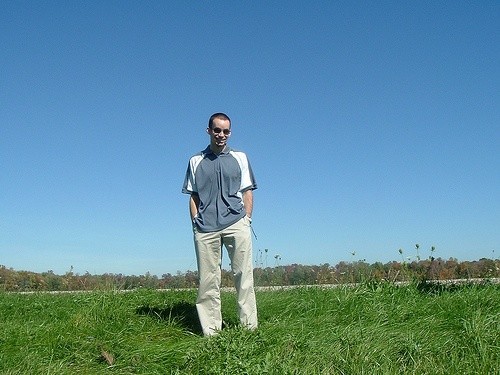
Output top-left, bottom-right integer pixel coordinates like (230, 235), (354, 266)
(211, 127), (231, 135)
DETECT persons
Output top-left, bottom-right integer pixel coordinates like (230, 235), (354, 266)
(181, 113), (258, 338)
